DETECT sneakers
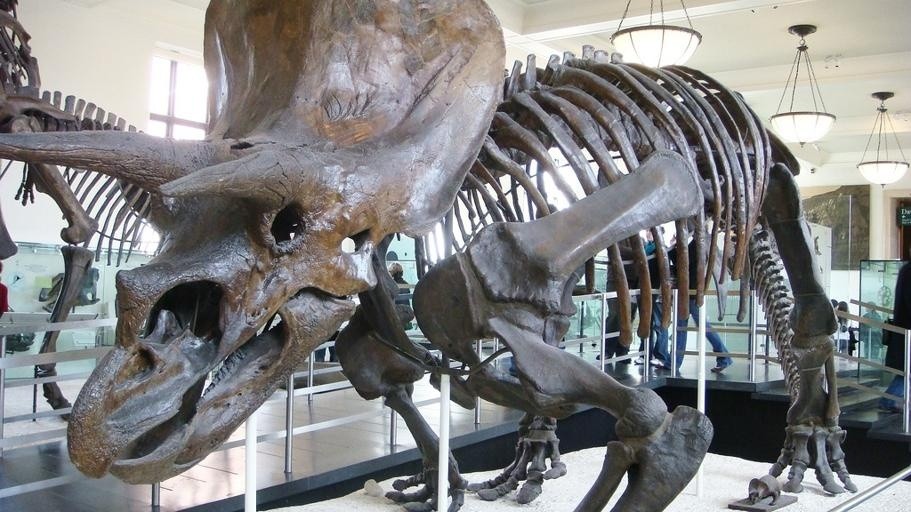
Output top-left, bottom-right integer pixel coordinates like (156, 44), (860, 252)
(596, 353), (665, 366)
(710, 363), (727, 372)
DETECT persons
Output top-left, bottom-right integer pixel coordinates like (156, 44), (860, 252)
(2, 260), (10, 321)
(876, 257), (911, 415)
(830, 297), (884, 361)
(389, 264), (412, 306)
(597, 233), (642, 364)
(636, 224), (672, 367)
(661, 217), (734, 373)
(314, 331), (344, 363)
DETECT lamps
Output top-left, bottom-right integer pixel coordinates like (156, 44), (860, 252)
(855, 92), (910, 189)
(609, 0), (703, 69)
(768, 24), (837, 148)
